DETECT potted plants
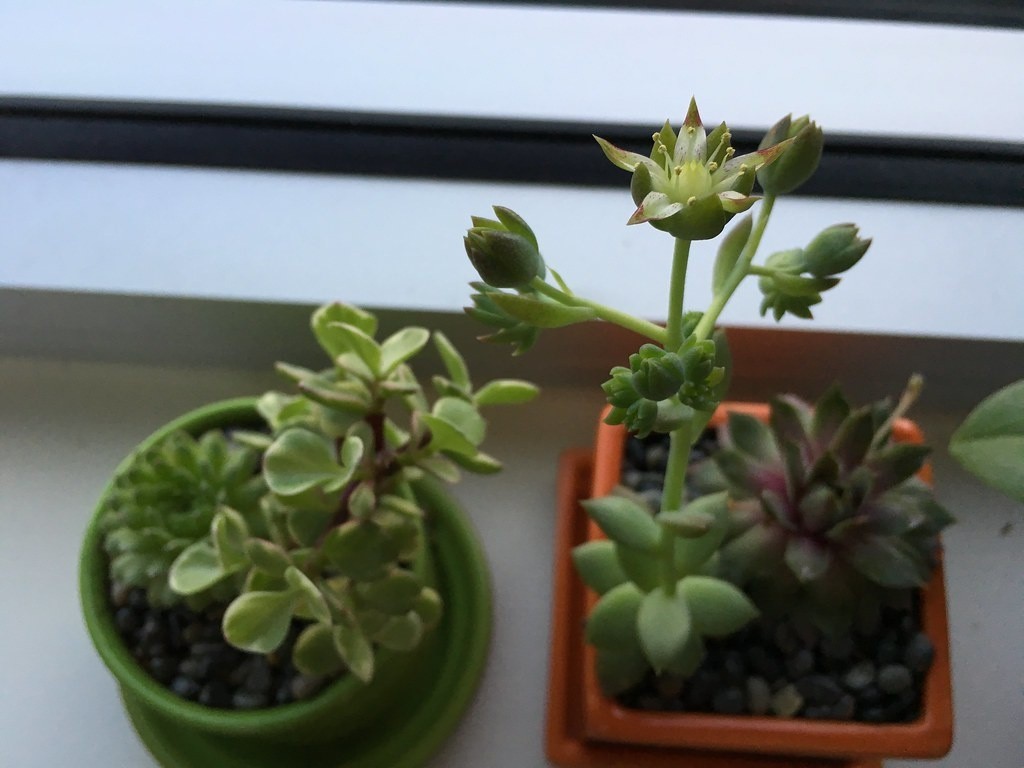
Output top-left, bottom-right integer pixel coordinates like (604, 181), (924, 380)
(79, 298), (541, 767)
(464, 95), (957, 767)
